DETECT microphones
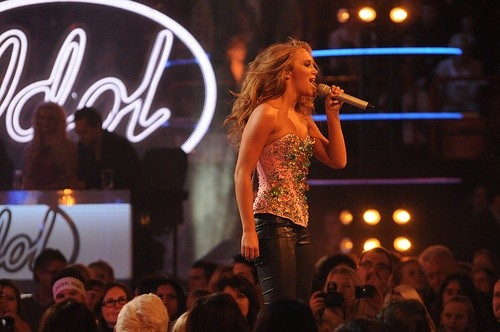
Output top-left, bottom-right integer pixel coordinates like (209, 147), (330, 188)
(316, 84), (374, 111)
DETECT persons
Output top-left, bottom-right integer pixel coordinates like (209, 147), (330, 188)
(224, 40), (348, 307)
(0, 0), (500, 332)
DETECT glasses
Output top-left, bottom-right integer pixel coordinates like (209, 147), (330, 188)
(101, 297), (129, 308)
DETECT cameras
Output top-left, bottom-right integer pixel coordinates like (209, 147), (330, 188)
(354, 285), (375, 299)
(317, 281), (344, 308)
